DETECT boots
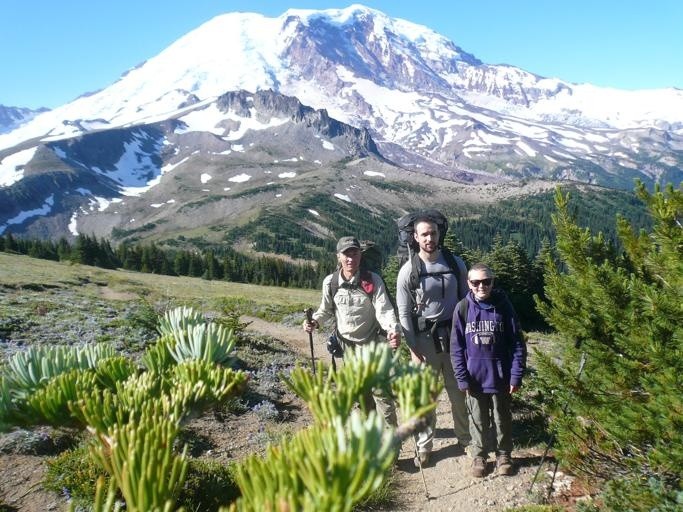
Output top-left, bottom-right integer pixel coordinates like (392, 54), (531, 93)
(458, 441), (487, 478)
(495, 451), (513, 475)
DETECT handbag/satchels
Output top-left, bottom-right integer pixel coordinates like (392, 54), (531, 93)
(412, 316), (432, 334)
(326, 334), (344, 358)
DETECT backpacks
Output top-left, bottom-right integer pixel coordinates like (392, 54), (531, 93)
(331, 240), (382, 300)
(398, 209), (462, 316)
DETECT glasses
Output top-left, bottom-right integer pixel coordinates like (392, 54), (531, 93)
(468, 277), (493, 287)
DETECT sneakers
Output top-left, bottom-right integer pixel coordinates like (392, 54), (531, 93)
(392, 457), (399, 468)
(413, 450), (429, 468)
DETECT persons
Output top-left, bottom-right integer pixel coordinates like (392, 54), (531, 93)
(448, 261), (527, 479)
(301, 236), (403, 472)
(393, 215), (475, 469)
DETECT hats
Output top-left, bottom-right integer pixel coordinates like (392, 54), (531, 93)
(336, 236), (360, 252)
